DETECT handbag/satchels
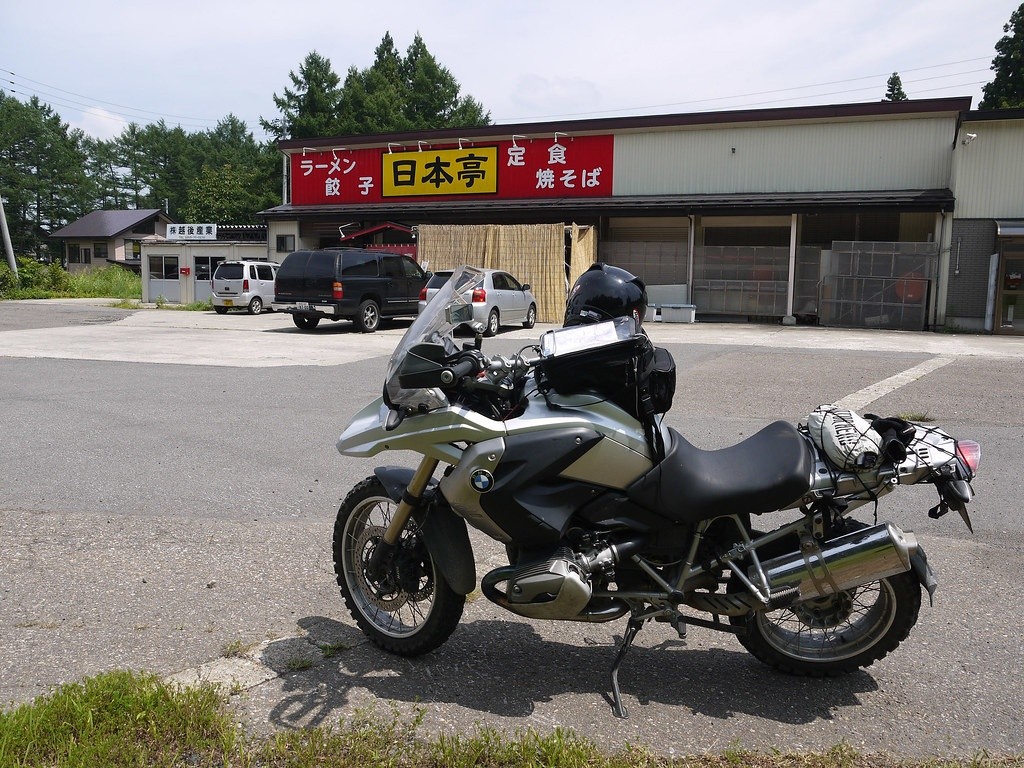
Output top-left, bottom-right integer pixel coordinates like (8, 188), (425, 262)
(534, 315), (676, 423)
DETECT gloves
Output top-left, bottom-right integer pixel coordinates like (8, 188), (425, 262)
(864, 413), (917, 464)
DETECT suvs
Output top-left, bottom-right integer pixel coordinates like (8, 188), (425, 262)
(210, 259), (284, 315)
(271, 247), (435, 334)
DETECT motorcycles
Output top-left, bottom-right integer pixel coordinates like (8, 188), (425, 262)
(330, 265), (984, 717)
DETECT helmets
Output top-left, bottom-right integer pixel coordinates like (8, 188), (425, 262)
(562, 263), (647, 333)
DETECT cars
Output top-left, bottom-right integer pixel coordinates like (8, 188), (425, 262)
(416, 268), (537, 337)
